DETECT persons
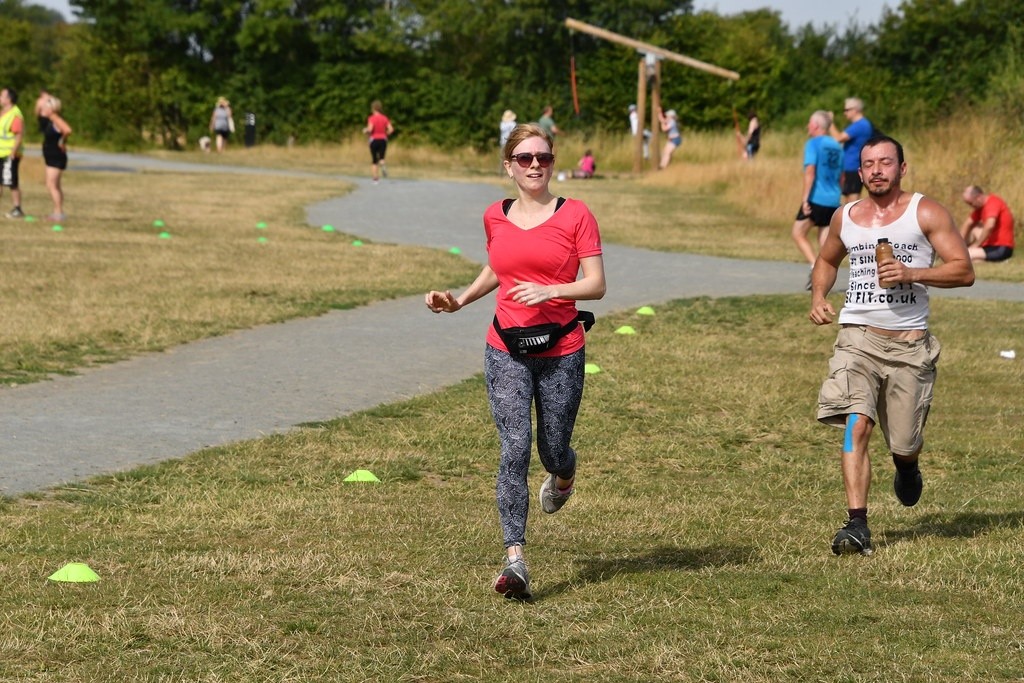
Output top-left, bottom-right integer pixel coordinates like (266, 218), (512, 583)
(35, 93), (71, 223)
(568, 150), (595, 178)
(198, 136), (211, 153)
(628, 105), (650, 158)
(500, 110), (517, 176)
(538, 106), (567, 138)
(961, 186), (1014, 263)
(362, 100), (394, 185)
(425, 123), (606, 600)
(792, 110), (846, 290)
(658, 107), (681, 169)
(828, 98), (872, 203)
(0, 87), (24, 219)
(745, 110), (760, 158)
(209, 97), (234, 152)
(809, 136), (975, 556)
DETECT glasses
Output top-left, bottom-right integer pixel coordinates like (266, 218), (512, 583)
(842, 106), (855, 112)
(509, 153), (554, 168)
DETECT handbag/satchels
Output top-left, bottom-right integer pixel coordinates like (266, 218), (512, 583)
(228, 112), (236, 134)
(493, 310), (595, 357)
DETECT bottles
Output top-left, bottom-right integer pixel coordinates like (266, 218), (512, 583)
(876, 238), (899, 289)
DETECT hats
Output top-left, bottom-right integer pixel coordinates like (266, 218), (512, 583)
(215, 96), (230, 107)
(502, 110), (516, 121)
(628, 104), (637, 112)
(664, 109), (679, 120)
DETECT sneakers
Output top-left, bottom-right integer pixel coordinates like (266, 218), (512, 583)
(832, 518), (872, 555)
(892, 454), (923, 507)
(539, 451), (577, 514)
(495, 542), (533, 603)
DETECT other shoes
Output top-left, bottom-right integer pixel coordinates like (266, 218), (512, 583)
(43, 212), (65, 223)
(806, 267), (814, 290)
(5, 209), (24, 219)
